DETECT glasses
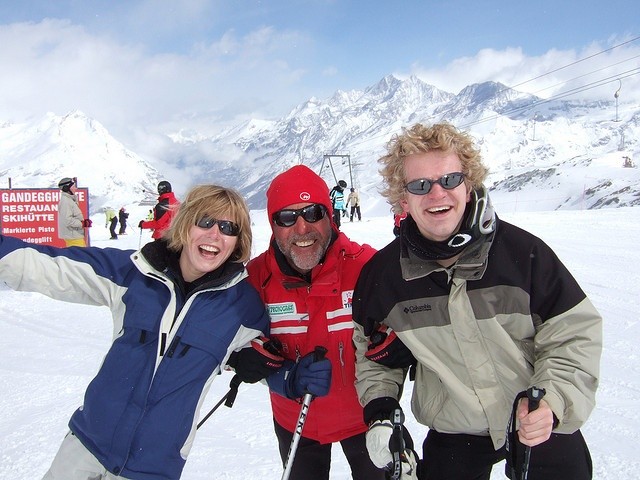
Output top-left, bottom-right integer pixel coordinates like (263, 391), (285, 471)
(404, 172), (465, 195)
(273, 203), (328, 227)
(58, 177), (77, 186)
(195, 213), (240, 236)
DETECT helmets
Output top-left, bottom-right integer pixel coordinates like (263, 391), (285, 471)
(59, 178), (75, 190)
(157, 181), (171, 195)
(337, 180), (347, 188)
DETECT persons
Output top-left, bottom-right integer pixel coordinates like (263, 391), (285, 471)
(241, 164), (401, 480)
(346, 188), (362, 223)
(102, 206), (119, 240)
(138, 181), (186, 248)
(327, 179), (349, 230)
(144, 207), (155, 233)
(351, 123), (602, 479)
(0, 184), (332, 479)
(57, 178), (92, 248)
(118, 207), (130, 235)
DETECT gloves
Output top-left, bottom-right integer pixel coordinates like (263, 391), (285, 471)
(366, 409), (419, 479)
(224, 348), (291, 408)
(267, 352), (332, 401)
(364, 324), (416, 380)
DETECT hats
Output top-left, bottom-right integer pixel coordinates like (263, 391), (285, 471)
(266, 164), (333, 231)
